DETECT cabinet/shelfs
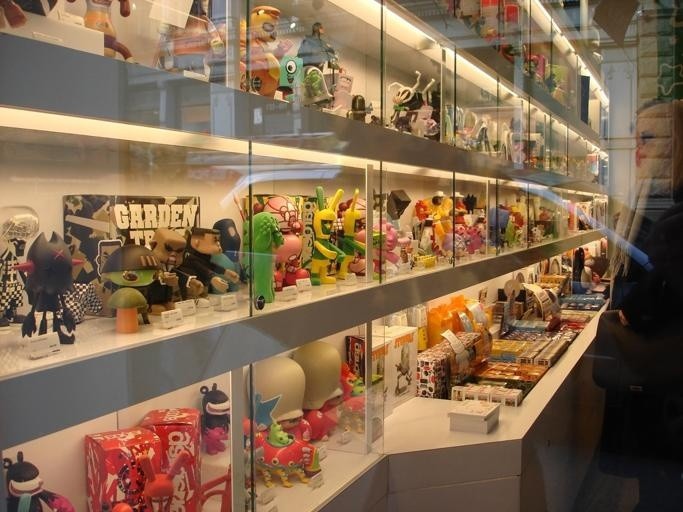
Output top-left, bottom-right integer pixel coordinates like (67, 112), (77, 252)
(0, 1), (611, 511)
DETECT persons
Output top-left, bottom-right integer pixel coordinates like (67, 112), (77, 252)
(0, 0), (443, 143)
(146, 188), (553, 315)
(245, 341), (344, 443)
(596, 98), (683, 510)
(449, 102), (541, 170)
(447, 0), (575, 111)
